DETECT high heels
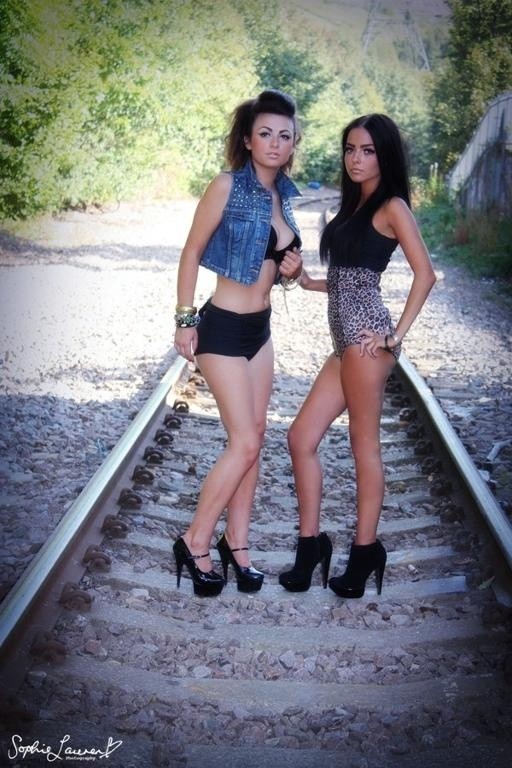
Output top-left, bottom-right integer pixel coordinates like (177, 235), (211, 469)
(172, 533), (264, 597)
(279, 532), (387, 598)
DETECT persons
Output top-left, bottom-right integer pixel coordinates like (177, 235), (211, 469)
(283, 109), (438, 599)
(173, 90), (304, 600)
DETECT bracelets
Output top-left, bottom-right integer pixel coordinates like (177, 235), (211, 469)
(383, 334), (392, 353)
(392, 335), (402, 344)
(174, 304), (198, 313)
(176, 314), (200, 327)
(279, 274), (302, 291)
